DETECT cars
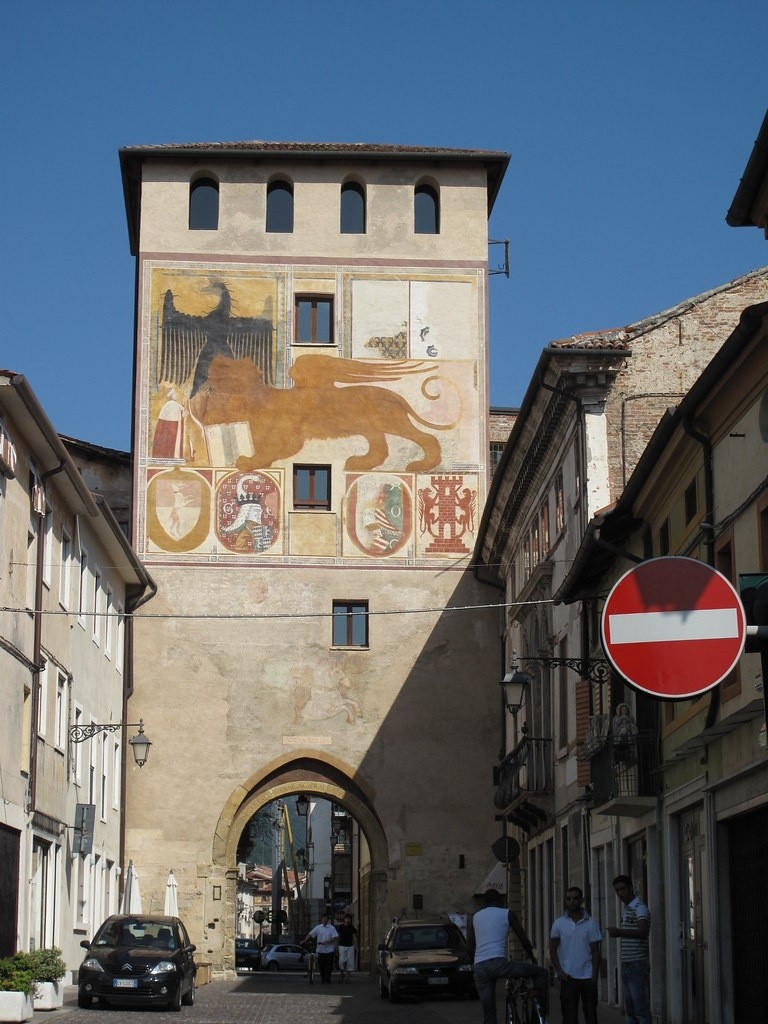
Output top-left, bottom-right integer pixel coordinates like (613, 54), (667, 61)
(234, 938), (262, 969)
(260, 944), (319, 973)
(76, 913), (197, 1011)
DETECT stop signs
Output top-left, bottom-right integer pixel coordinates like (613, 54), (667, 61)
(600, 554), (748, 702)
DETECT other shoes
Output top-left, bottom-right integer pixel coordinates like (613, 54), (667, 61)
(321, 977), (332, 984)
(338, 974), (344, 983)
(346, 976), (351, 984)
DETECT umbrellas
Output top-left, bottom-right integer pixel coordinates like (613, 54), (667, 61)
(119, 858), (144, 936)
(163, 870), (184, 941)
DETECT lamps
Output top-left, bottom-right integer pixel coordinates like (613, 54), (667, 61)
(295, 793), (309, 816)
(323, 876), (334, 888)
(70, 717), (153, 769)
(496, 652), (607, 717)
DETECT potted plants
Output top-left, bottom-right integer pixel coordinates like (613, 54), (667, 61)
(26, 945), (67, 1011)
(0, 950), (45, 1023)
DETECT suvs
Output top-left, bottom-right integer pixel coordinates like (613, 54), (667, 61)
(376, 913), (475, 1003)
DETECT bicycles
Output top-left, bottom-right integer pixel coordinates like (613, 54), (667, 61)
(299, 941), (322, 984)
(502, 955), (548, 1024)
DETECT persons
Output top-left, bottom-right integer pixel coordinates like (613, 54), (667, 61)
(606, 875), (652, 1024)
(299, 914), (338, 984)
(550, 887), (602, 1024)
(467, 889), (538, 1024)
(612, 702), (639, 743)
(337, 914), (359, 984)
